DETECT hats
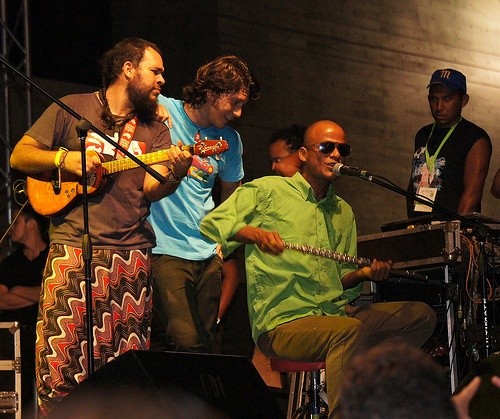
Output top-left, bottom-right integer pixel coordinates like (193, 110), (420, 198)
(427, 69), (466, 96)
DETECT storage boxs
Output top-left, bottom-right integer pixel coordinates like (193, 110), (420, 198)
(356, 221), (470, 396)
(0, 321), (22, 419)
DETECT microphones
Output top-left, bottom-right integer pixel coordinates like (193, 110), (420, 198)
(332, 163), (371, 177)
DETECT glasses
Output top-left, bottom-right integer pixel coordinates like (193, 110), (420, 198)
(305, 141), (353, 157)
(271, 149), (299, 163)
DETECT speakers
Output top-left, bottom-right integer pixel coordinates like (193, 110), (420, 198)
(48, 349), (285, 419)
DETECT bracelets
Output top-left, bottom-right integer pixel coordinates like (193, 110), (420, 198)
(168, 171), (184, 185)
(54, 147), (69, 171)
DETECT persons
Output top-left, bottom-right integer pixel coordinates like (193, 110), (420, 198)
(406, 68), (500, 220)
(153, 54), (311, 353)
(200, 119), (438, 419)
(332, 338), (500, 419)
(0, 202), (50, 419)
(8, 37), (194, 419)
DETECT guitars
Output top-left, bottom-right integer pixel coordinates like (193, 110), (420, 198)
(27, 131), (229, 219)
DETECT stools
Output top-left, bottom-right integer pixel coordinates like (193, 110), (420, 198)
(271, 356), (326, 418)
